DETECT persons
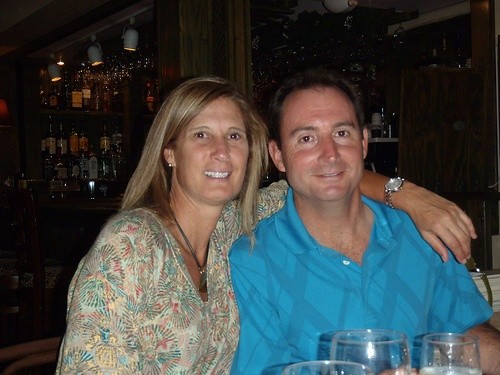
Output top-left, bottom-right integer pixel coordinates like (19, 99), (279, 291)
(229, 74), (500, 375)
(55, 77), (476, 375)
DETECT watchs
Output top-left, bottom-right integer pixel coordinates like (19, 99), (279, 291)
(384, 177), (406, 208)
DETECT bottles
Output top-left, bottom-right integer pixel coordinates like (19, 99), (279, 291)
(371, 95), (398, 138)
(81, 83), (91, 112)
(49, 85), (58, 110)
(9, 273), (19, 288)
(56, 123), (68, 159)
(69, 154), (80, 184)
(17, 168), (27, 193)
(91, 87), (121, 112)
(70, 86), (83, 111)
(89, 144), (121, 181)
(421, 33), (464, 67)
(69, 121), (78, 156)
(112, 123), (123, 154)
(80, 149), (89, 180)
(41, 112), (55, 179)
(79, 121), (88, 155)
(100, 123), (111, 154)
(143, 81), (155, 115)
(55, 146), (66, 179)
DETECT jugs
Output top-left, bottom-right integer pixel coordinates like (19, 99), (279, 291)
(71, 169), (113, 198)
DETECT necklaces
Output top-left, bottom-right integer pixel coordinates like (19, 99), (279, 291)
(169, 207), (209, 292)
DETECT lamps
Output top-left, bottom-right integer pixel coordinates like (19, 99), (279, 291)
(88, 36), (104, 68)
(120, 18), (140, 51)
(47, 63), (62, 81)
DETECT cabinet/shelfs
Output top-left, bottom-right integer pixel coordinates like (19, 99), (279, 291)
(17, 1), (177, 338)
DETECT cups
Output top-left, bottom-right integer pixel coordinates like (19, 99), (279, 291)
(330, 330), (410, 375)
(420, 333), (482, 375)
(21, 272), (34, 288)
(281, 361), (372, 375)
